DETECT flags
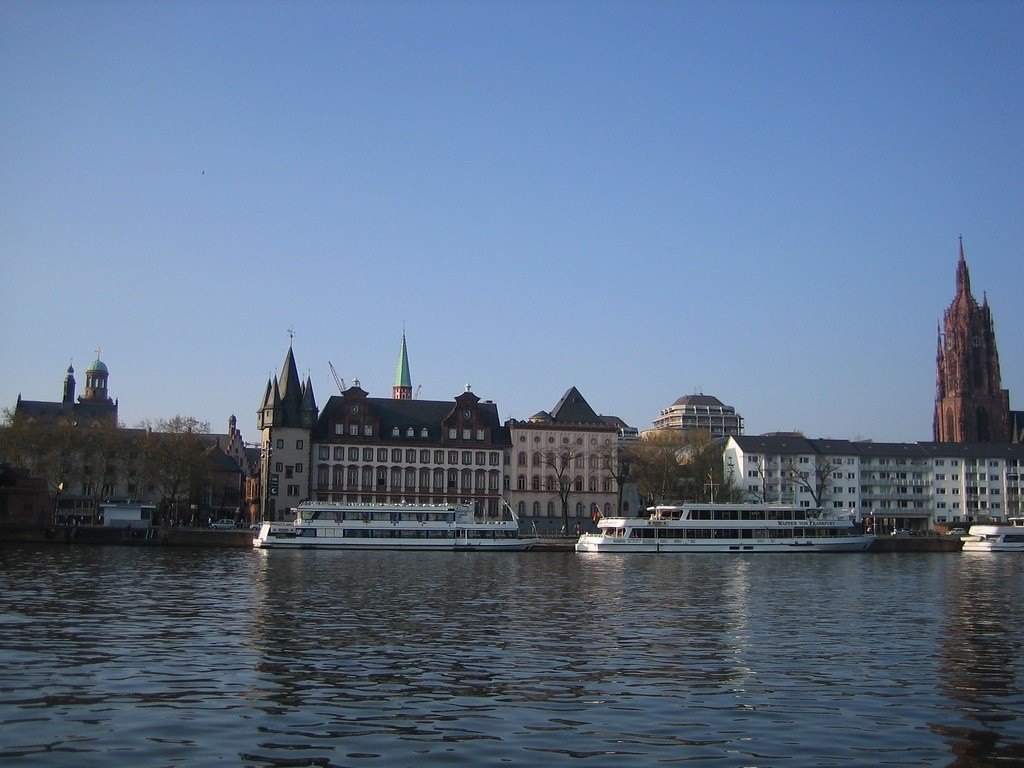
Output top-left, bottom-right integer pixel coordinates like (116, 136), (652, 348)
(593, 506), (600, 521)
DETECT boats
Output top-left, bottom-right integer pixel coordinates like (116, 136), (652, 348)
(574, 503), (878, 554)
(252, 500), (540, 551)
(960, 516), (1024, 552)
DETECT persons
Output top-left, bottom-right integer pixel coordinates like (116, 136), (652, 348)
(65, 512), (68, 525)
(162, 515), (244, 530)
(532, 521), (580, 536)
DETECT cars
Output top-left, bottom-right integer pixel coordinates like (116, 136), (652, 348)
(945, 528), (967, 535)
(249, 522), (260, 531)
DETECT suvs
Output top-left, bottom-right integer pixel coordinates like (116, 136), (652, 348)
(210, 519), (237, 529)
